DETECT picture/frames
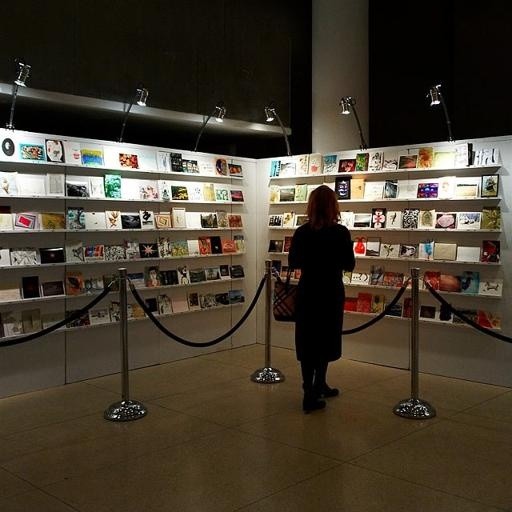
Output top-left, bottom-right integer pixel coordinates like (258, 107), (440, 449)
(395, 152), (420, 171)
(480, 173), (501, 197)
(153, 213), (172, 229)
(170, 205), (187, 229)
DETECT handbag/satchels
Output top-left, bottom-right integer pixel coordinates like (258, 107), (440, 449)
(274, 282), (298, 322)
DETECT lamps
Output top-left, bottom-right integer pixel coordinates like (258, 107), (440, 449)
(6, 55), (31, 131)
(190, 98), (231, 155)
(119, 78), (150, 142)
(264, 105), (294, 159)
(423, 79), (456, 142)
(335, 95), (367, 147)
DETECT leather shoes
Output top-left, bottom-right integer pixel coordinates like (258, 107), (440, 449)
(303, 397), (326, 411)
(313, 382), (338, 397)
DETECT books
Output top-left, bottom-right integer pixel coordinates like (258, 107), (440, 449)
(269, 143), (501, 331)
(2, 130), (245, 338)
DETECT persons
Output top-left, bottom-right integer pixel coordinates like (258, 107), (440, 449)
(286, 184), (358, 414)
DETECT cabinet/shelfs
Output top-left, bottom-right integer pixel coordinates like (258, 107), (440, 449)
(63, 155), (256, 348)
(267, 162), (512, 334)
(0, 157), (64, 343)
